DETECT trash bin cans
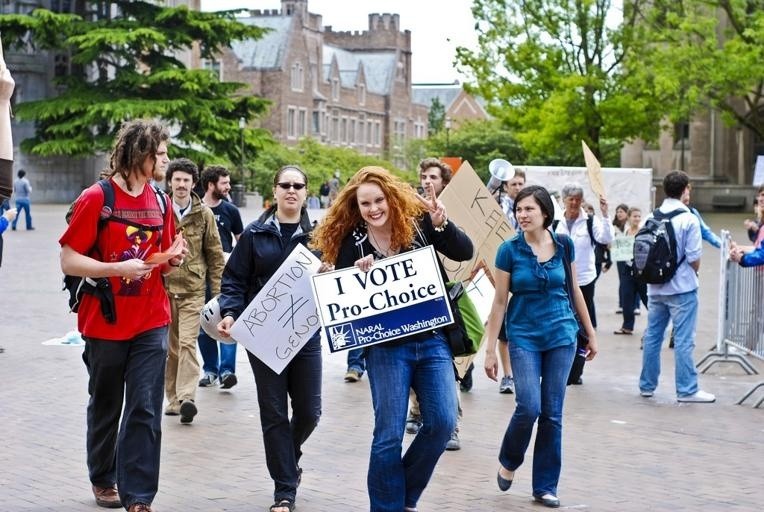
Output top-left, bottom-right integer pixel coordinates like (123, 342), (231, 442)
(231, 184), (246, 207)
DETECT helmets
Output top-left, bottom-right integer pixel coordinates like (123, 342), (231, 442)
(198, 293), (238, 346)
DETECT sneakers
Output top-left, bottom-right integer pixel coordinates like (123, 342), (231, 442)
(198, 371), (220, 387)
(406, 412), (422, 434)
(91, 482), (125, 509)
(460, 361), (475, 392)
(343, 369), (360, 382)
(445, 429), (461, 451)
(676, 389), (716, 404)
(615, 307), (625, 314)
(180, 399), (197, 424)
(219, 370), (237, 389)
(640, 390), (654, 398)
(633, 307), (641, 316)
(499, 376), (515, 394)
(165, 403), (182, 416)
(126, 502), (153, 512)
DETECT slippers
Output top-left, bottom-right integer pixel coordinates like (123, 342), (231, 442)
(613, 328), (633, 335)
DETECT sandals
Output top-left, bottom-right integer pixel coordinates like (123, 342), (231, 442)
(294, 464), (301, 488)
(270, 498), (295, 512)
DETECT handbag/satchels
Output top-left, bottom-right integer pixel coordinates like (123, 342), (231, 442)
(565, 330), (590, 387)
(437, 280), (487, 357)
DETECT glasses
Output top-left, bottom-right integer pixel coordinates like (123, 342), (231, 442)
(274, 182), (307, 190)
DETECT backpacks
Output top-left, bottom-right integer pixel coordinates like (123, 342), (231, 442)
(550, 214), (613, 282)
(631, 208), (687, 284)
(63, 177), (169, 325)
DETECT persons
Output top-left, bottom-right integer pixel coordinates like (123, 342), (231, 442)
(0, 58), (34, 274)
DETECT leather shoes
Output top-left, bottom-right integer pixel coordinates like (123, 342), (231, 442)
(496, 463), (515, 492)
(532, 490), (561, 508)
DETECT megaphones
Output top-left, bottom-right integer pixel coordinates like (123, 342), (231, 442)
(484, 159), (514, 194)
